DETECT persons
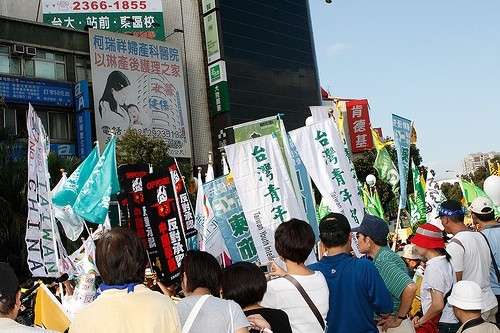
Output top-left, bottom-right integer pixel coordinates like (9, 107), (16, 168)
(0, 195), (500, 333)
(98, 70), (146, 129)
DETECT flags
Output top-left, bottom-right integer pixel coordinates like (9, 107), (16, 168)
(24, 101), (60, 278)
(50, 105), (500, 287)
(33, 280), (73, 333)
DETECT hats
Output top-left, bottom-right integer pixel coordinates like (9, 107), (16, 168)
(351, 215), (389, 240)
(319, 213), (350, 233)
(401, 244), (424, 260)
(447, 280), (483, 310)
(0, 262), (19, 301)
(467, 197), (494, 214)
(408, 223), (445, 249)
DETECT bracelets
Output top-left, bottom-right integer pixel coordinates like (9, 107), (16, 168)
(395, 312), (407, 320)
(418, 319), (423, 327)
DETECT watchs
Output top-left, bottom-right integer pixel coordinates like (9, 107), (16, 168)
(260, 327), (273, 333)
